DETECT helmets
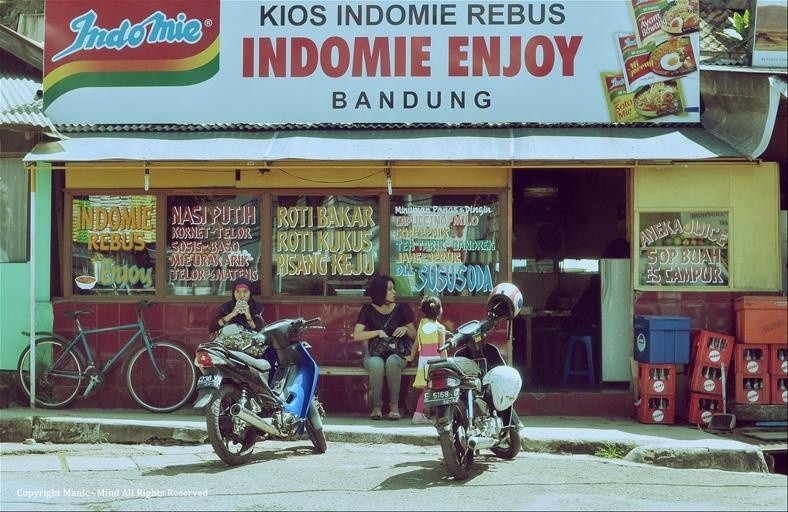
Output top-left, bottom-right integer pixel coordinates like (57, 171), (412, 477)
(486, 281), (524, 318)
(482, 365), (524, 411)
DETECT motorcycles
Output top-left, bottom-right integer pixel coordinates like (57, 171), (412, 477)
(192, 317), (328, 465)
(423, 302), (524, 481)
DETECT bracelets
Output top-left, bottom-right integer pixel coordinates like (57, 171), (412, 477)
(221, 317), (227, 324)
(246, 318), (254, 322)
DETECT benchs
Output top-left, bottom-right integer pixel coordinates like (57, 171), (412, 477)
(316, 365), (416, 375)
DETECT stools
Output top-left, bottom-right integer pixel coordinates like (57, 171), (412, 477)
(561, 334), (596, 385)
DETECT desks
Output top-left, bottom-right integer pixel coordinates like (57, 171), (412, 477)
(520, 310), (571, 385)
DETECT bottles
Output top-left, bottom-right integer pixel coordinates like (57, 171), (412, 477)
(660, 369), (666, 380)
(704, 366), (711, 378)
(760, 380), (763, 388)
(745, 349), (752, 360)
(652, 400), (657, 408)
(653, 369), (658, 380)
(709, 337), (715, 349)
(752, 351), (756, 359)
(780, 351), (784, 360)
(716, 339), (722, 349)
(715, 338), (718, 346)
(238, 300), (246, 314)
(658, 398), (665, 410)
(701, 399), (708, 409)
(780, 379), (785, 389)
(711, 368), (718, 379)
(754, 379), (760, 388)
(709, 400), (715, 410)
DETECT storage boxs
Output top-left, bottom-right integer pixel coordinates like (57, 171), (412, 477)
(634, 315), (691, 364)
(735, 295), (788, 343)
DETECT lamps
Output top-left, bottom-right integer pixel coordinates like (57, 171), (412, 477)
(385, 161), (393, 195)
(144, 160), (150, 191)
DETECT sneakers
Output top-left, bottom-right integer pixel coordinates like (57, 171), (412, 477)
(410, 410), (433, 424)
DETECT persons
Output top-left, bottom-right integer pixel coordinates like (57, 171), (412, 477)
(355, 276), (417, 420)
(207, 276), (279, 385)
(406, 297), (448, 424)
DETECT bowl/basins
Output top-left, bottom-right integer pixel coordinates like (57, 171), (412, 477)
(648, 37), (696, 77)
(632, 80), (679, 118)
(74, 274), (98, 289)
(658, 0), (699, 33)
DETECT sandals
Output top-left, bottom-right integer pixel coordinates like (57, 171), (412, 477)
(370, 411), (383, 420)
(388, 411), (401, 419)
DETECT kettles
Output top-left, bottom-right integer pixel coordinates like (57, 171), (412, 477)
(744, 379), (752, 388)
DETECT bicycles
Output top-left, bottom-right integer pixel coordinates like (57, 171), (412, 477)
(15, 300), (194, 414)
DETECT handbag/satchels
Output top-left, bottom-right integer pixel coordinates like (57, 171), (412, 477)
(208, 321), (271, 358)
(369, 332), (413, 357)
